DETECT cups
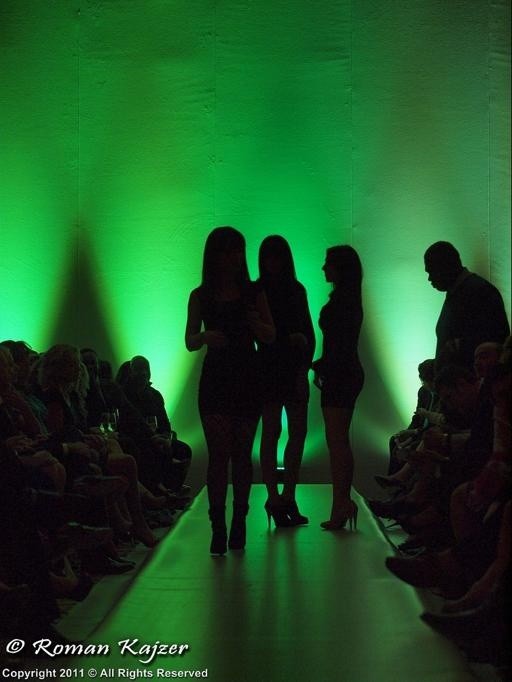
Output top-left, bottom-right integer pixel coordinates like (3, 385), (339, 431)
(166, 432), (174, 444)
(145, 415), (157, 432)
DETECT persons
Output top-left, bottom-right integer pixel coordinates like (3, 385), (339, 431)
(424, 240), (510, 368)
(310, 246), (364, 531)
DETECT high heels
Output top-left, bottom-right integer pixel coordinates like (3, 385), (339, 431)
(320, 500), (359, 531)
(143, 481), (178, 510)
(129, 523), (160, 548)
(208, 502), (249, 556)
(265, 497), (308, 529)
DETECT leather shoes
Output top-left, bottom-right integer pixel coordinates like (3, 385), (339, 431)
(85, 548), (137, 575)
(375, 474), (510, 665)
